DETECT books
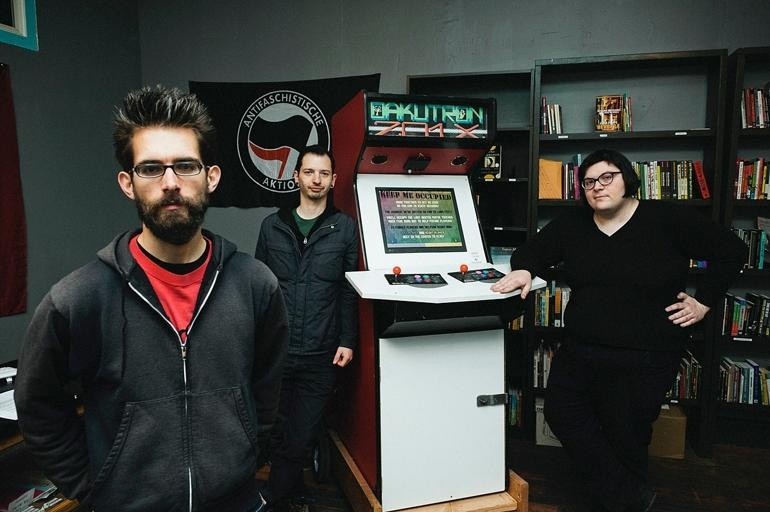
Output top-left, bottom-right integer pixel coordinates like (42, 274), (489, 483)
(666, 349), (702, 401)
(722, 292), (768, 336)
(509, 309), (525, 330)
(533, 341), (553, 388)
(720, 357), (768, 405)
(542, 97), (563, 134)
(538, 154), (709, 200)
(730, 229), (766, 269)
(735, 157), (768, 199)
(534, 282), (571, 328)
(596, 88), (632, 132)
(507, 388), (522, 426)
(741, 88), (769, 129)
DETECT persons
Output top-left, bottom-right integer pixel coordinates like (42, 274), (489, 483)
(491, 149), (749, 511)
(253, 146), (359, 512)
(12, 84), (290, 511)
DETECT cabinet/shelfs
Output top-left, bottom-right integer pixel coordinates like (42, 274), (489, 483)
(715, 42), (770, 456)
(403, 69), (537, 442)
(532, 47), (729, 445)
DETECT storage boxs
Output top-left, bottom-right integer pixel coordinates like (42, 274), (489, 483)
(532, 397), (563, 449)
(648, 399), (688, 462)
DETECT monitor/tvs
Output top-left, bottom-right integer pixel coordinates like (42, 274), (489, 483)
(375, 186), (468, 254)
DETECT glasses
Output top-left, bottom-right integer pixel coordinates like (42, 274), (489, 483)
(580, 171), (621, 190)
(128, 163), (205, 176)
